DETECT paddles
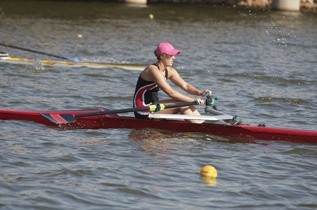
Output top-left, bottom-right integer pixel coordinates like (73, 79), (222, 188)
(38, 99), (205, 124)
(0, 42), (79, 63)
(204, 90), (219, 112)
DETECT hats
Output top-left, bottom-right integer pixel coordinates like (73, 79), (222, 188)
(156, 42), (180, 56)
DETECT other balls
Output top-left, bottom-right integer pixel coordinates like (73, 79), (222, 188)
(200, 164), (219, 178)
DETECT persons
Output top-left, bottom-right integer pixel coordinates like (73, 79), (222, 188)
(132, 41), (212, 125)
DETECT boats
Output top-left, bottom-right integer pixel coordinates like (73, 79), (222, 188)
(0, 108), (316, 143)
